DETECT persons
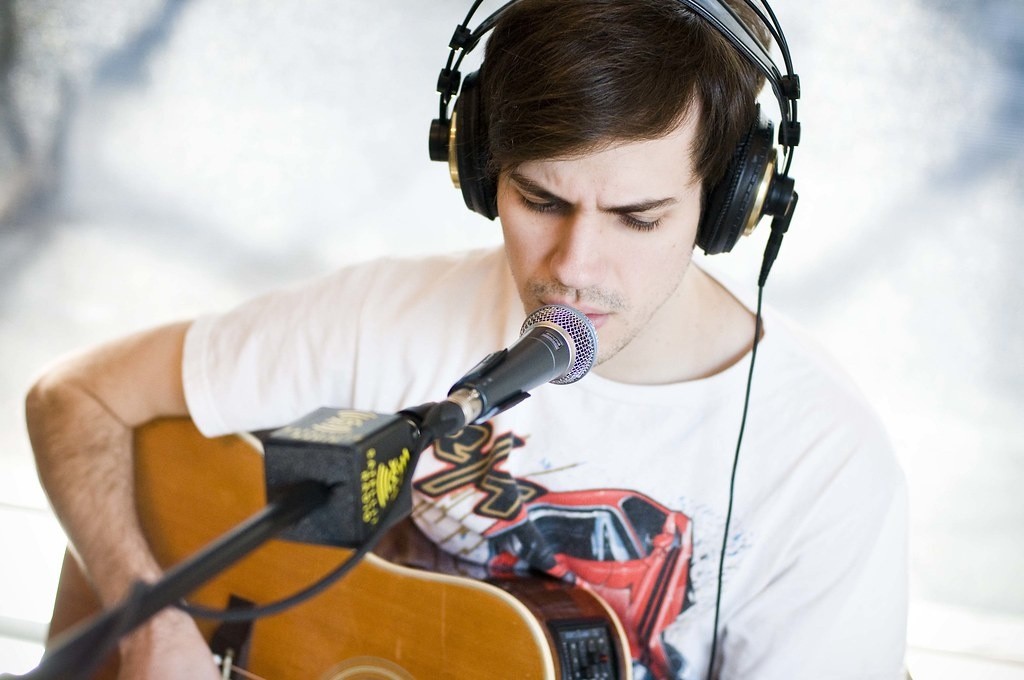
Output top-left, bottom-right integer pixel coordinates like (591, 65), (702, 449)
(27, 0), (907, 680)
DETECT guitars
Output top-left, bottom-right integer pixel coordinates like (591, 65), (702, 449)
(45, 414), (638, 678)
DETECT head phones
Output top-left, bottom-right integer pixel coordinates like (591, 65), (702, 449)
(427, 0), (801, 288)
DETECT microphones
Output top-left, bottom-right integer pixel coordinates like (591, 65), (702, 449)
(424, 303), (599, 439)
